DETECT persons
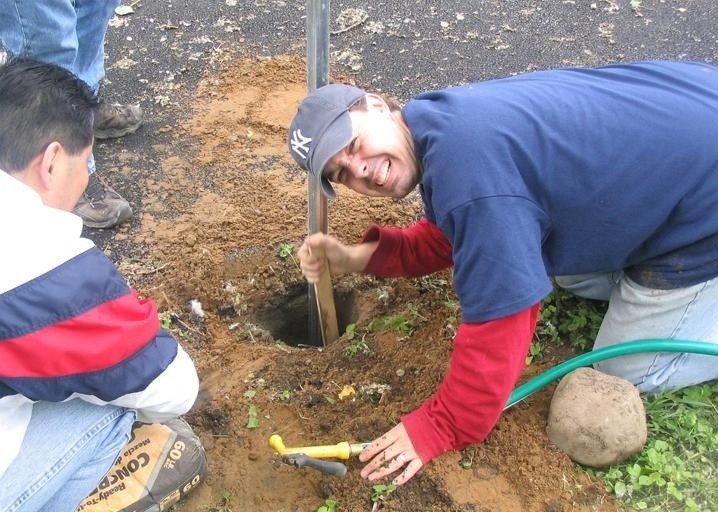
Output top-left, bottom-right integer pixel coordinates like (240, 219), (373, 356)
(0, 0), (143, 228)
(286, 60), (717, 486)
(0, 60), (201, 512)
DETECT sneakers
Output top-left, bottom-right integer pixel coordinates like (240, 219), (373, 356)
(69, 169), (133, 230)
(91, 97), (144, 140)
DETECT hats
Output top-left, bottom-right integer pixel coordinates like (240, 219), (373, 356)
(288, 83), (364, 200)
